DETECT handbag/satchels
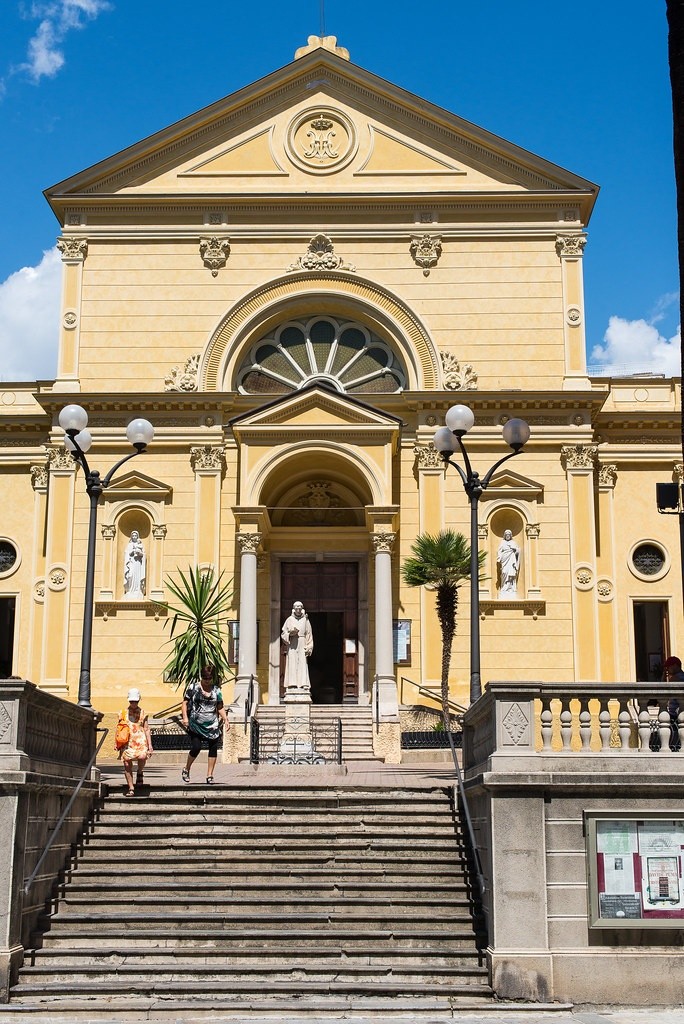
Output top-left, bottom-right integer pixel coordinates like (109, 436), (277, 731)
(115, 708), (129, 751)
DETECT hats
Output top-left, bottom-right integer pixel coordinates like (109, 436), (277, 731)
(127, 688), (141, 701)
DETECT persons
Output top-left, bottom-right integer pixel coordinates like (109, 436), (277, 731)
(662, 657), (684, 711)
(123, 531), (145, 594)
(114, 688), (152, 797)
(282, 601), (313, 689)
(497, 529), (519, 591)
(181, 666), (230, 784)
(615, 859), (623, 870)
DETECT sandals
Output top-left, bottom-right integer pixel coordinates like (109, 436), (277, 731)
(126, 789), (135, 797)
(136, 770), (143, 786)
(182, 767), (190, 782)
(206, 777), (215, 784)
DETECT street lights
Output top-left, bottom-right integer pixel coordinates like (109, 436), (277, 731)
(56, 404), (155, 706)
(433, 402), (532, 702)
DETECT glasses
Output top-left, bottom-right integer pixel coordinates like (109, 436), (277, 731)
(202, 677), (212, 680)
